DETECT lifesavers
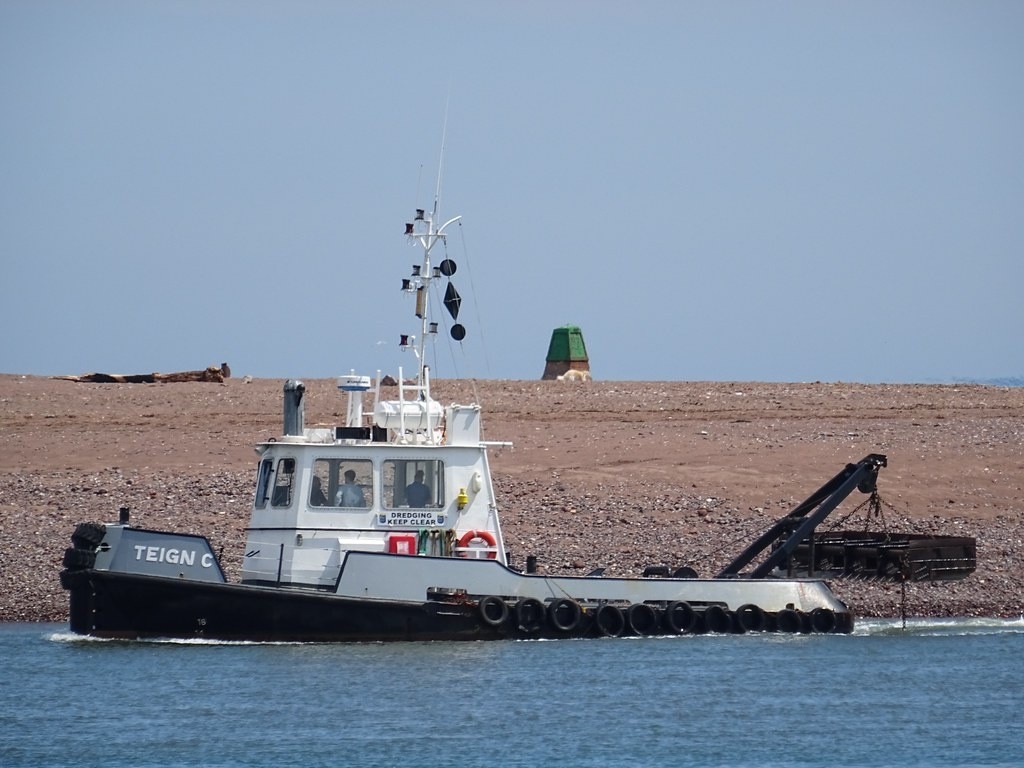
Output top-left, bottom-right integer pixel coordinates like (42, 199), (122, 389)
(479, 595), (854, 637)
(458, 530), (497, 558)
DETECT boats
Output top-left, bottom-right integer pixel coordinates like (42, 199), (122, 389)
(59, 95), (889, 641)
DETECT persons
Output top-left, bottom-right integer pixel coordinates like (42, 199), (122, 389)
(333, 469), (366, 508)
(310, 475), (327, 506)
(403, 469), (433, 509)
